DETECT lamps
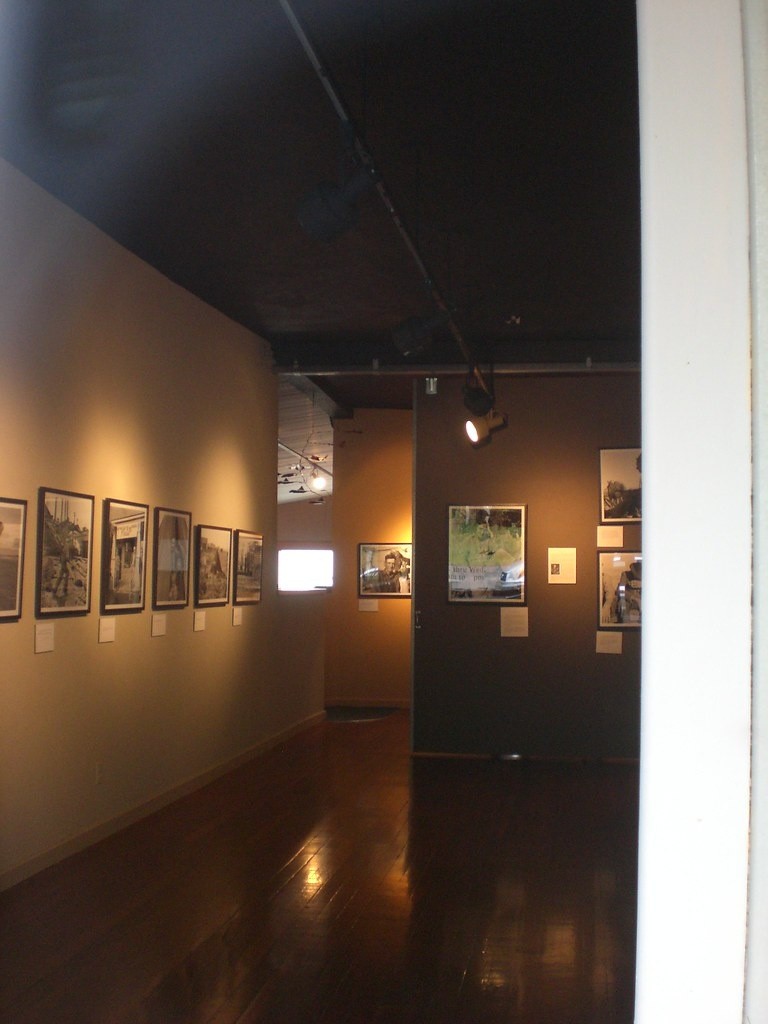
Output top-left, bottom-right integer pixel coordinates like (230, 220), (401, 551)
(466, 364), (508, 443)
(277, 391), (363, 504)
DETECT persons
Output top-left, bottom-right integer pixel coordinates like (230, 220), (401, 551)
(166, 539), (186, 600)
(601, 561), (641, 625)
(475, 508), (495, 555)
(52, 538), (73, 596)
(370, 554), (400, 593)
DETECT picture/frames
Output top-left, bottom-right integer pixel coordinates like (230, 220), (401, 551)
(357, 542), (413, 599)
(235, 530), (264, 605)
(195, 524), (232, 605)
(102, 499), (150, 613)
(444, 502), (528, 607)
(33, 487), (95, 616)
(0, 497), (28, 620)
(596, 446), (642, 525)
(597, 550), (641, 632)
(152, 507), (192, 608)
